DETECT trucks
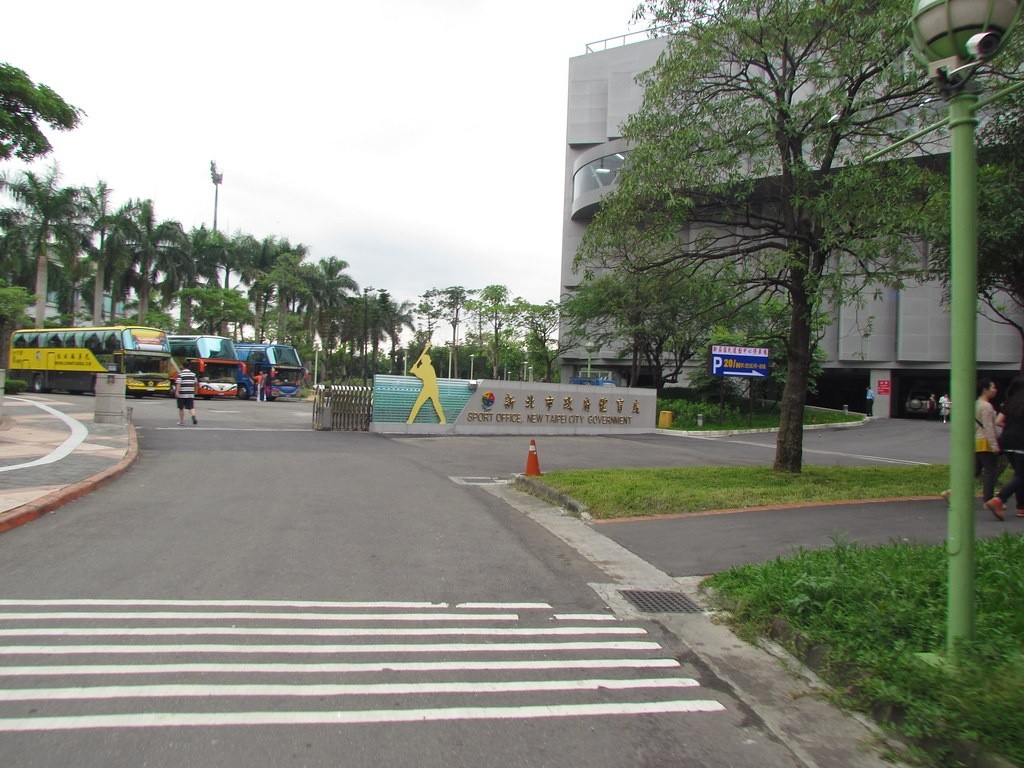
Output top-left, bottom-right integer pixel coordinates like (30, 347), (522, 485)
(572, 378), (618, 387)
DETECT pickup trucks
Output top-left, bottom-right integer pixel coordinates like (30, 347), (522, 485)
(906, 396), (933, 416)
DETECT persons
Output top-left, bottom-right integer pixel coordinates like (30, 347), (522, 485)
(866, 386), (874, 416)
(250, 369), (270, 402)
(174, 360), (198, 426)
(927, 390), (950, 423)
(974, 375), (1024, 520)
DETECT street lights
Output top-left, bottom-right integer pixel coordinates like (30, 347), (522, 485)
(208, 159), (223, 239)
(585, 340), (595, 379)
(469, 353), (476, 380)
(523, 361), (528, 380)
(902, 0), (1022, 665)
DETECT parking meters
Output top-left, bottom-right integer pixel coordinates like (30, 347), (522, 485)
(709, 344), (769, 376)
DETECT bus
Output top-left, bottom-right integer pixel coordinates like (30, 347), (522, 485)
(167, 335), (309, 403)
(9, 325), (175, 398)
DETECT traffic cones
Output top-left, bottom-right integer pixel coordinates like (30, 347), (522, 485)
(524, 439), (541, 477)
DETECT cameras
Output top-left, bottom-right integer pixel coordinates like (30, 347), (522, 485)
(966, 32), (1001, 59)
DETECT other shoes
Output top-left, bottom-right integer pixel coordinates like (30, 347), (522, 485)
(986, 498), (1005, 520)
(942, 490), (951, 503)
(983, 502), (1007, 510)
(177, 421), (184, 425)
(1017, 508), (1024, 517)
(192, 416), (197, 425)
